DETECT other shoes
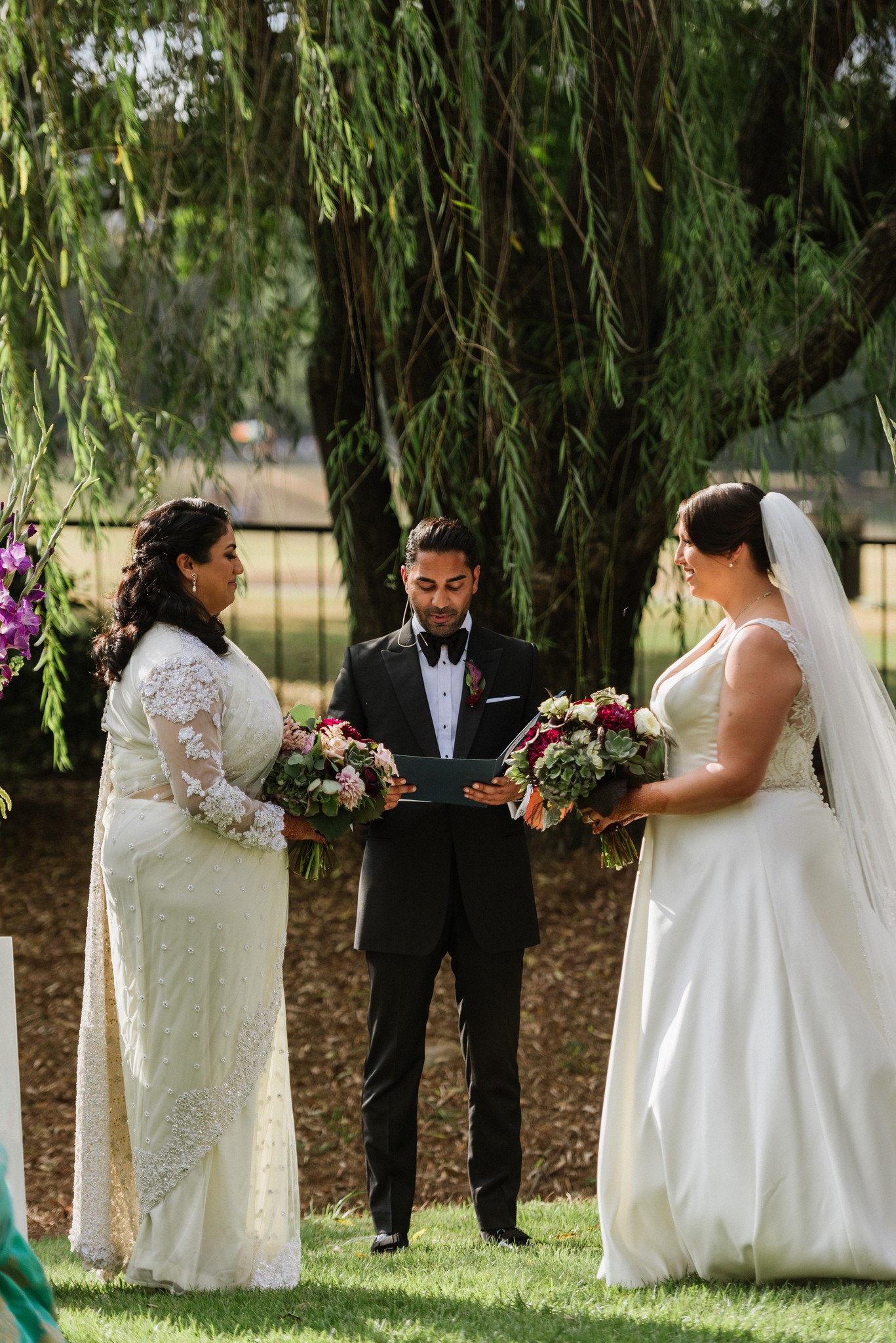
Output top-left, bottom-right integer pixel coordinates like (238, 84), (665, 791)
(481, 1227), (535, 1250)
(371, 1231), (408, 1255)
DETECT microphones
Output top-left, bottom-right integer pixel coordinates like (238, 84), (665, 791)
(407, 595), (430, 647)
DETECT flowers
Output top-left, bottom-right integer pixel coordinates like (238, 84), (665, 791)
(505, 685), (671, 872)
(261, 704), (398, 881)
(462, 659), (486, 708)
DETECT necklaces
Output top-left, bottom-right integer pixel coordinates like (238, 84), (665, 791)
(709, 584), (778, 647)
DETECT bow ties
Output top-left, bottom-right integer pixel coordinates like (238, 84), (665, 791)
(417, 628), (469, 667)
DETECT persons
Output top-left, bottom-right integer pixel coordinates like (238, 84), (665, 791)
(312, 518), (559, 1257)
(560, 480), (896, 1288)
(73, 495), (334, 1296)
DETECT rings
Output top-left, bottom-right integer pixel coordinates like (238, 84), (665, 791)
(586, 813), (598, 824)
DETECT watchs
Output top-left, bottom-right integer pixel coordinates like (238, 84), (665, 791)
(511, 788), (527, 803)
